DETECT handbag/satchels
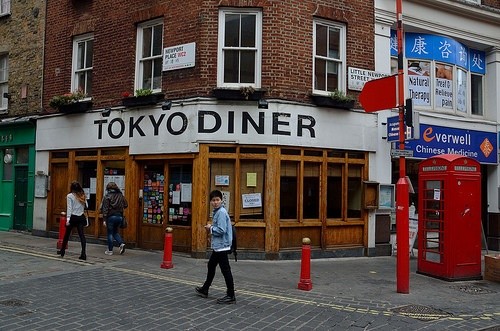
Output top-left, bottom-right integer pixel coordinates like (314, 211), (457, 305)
(119, 217), (127, 229)
(228, 225), (236, 254)
(83, 218), (89, 228)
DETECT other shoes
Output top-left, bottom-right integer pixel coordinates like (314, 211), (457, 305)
(105, 251), (113, 256)
(218, 295), (238, 304)
(195, 286), (208, 298)
(120, 243), (126, 254)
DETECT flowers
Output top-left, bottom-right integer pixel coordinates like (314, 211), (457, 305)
(121, 91), (133, 97)
(64, 86), (86, 101)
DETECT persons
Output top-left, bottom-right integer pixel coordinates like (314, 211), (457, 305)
(103, 182), (128, 255)
(194, 189), (237, 305)
(57, 182), (89, 261)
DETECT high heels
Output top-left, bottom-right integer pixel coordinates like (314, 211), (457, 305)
(57, 248), (65, 257)
(79, 254), (86, 260)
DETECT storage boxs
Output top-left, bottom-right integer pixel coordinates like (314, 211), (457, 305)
(484, 254), (500, 282)
